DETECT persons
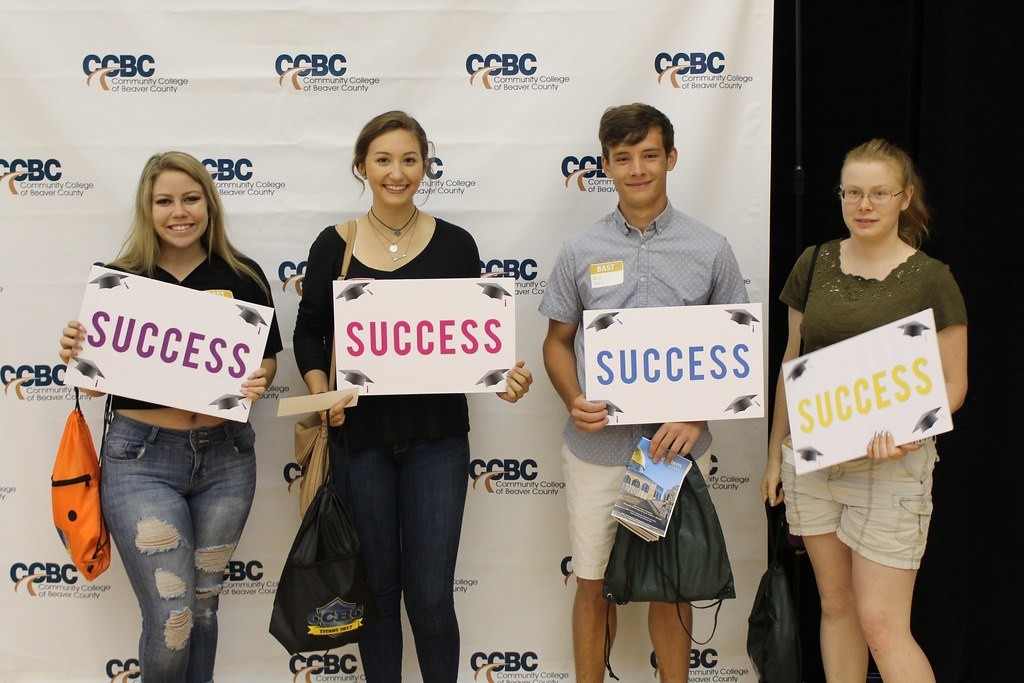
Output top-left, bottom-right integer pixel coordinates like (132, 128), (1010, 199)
(537, 104), (751, 683)
(58, 150), (284, 683)
(760, 139), (967, 683)
(292, 109), (535, 683)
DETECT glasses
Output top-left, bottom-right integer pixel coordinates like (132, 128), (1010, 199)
(839, 185), (904, 205)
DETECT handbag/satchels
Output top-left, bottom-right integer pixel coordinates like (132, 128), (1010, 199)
(293, 409), (329, 524)
(601, 437), (736, 603)
(268, 410), (381, 655)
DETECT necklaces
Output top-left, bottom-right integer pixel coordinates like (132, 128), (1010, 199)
(367, 206), (420, 261)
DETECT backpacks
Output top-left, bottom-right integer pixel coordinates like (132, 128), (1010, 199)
(747, 481), (802, 683)
(51, 387), (111, 583)
